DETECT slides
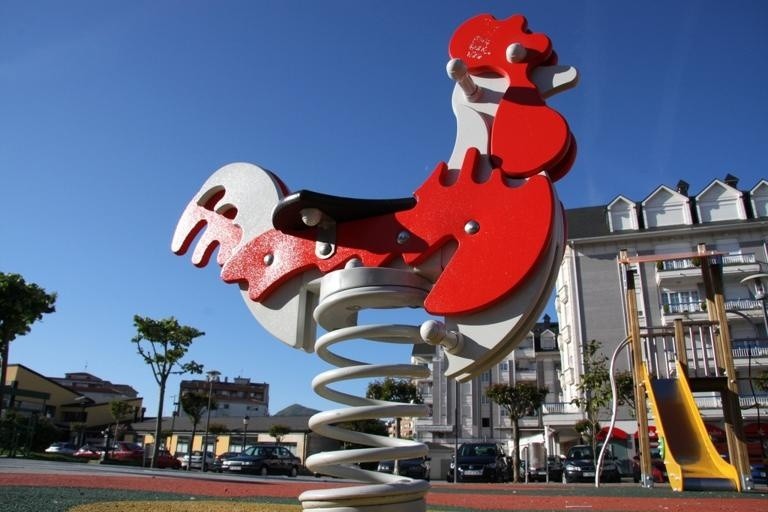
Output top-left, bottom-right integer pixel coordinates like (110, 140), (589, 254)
(642, 360), (741, 495)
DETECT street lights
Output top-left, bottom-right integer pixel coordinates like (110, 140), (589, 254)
(241, 412), (252, 450)
(101, 424), (115, 462)
(201, 370), (221, 471)
(167, 411), (178, 465)
(740, 273), (768, 337)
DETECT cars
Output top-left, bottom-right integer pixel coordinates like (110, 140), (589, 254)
(717, 453), (767, 485)
(46, 438), (303, 477)
